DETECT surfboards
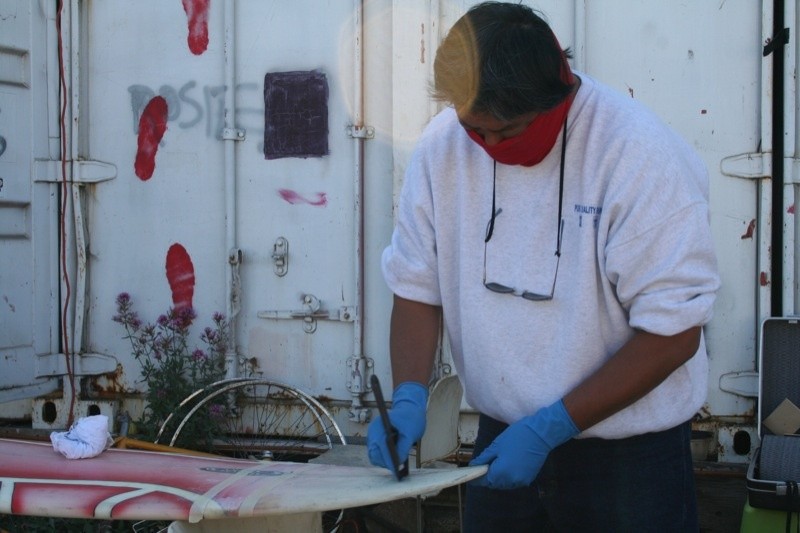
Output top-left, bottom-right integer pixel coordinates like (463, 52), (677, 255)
(0, 438), (489, 520)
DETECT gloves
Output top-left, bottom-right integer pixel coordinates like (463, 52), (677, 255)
(367, 382), (430, 476)
(469, 397), (583, 492)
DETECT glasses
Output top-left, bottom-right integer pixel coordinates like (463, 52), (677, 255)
(483, 207), (565, 301)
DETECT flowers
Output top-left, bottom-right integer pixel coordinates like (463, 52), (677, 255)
(112, 293), (232, 422)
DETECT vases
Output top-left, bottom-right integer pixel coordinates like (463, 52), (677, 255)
(163, 416), (210, 446)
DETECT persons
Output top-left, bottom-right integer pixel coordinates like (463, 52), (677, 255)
(367, 0), (721, 533)
